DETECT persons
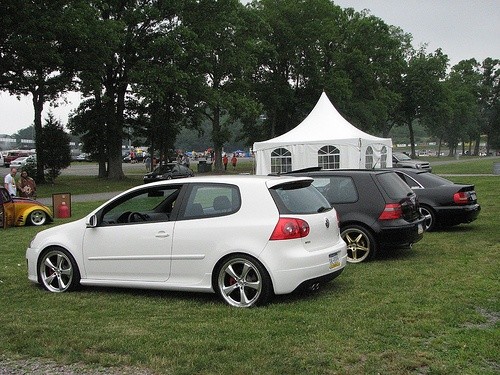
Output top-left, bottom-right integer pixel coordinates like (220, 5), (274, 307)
(231, 155), (237, 171)
(143, 155), (156, 173)
(223, 154), (228, 170)
(17, 171), (37, 201)
(4, 167), (18, 197)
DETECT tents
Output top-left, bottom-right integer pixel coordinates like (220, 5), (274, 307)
(253, 91), (394, 175)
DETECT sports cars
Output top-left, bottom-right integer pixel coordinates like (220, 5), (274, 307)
(0, 185), (54, 229)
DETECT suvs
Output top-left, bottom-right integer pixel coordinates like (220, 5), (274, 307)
(24, 175), (350, 309)
(266, 166), (427, 264)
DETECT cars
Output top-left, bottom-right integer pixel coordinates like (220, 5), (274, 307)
(392, 152), (432, 173)
(0, 149), (46, 172)
(76, 153), (94, 162)
(185, 151), (228, 158)
(123, 154), (131, 163)
(418, 152), (431, 157)
(143, 162), (195, 184)
(372, 167), (481, 232)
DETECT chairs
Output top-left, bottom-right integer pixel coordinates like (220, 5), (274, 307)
(186, 204), (205, 216)
(213, 196), (231, 211)
